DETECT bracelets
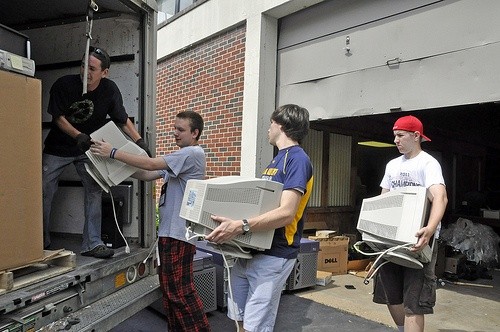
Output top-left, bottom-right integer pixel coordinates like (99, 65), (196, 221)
(109, 148), (117, 160)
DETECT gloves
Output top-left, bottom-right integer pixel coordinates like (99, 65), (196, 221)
(75, 133), (94, 152)
(137, 138), (152, 158)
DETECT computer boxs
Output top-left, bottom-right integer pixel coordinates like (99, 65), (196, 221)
(0, 50), (35, 77)
(100, 197), (126, 250)
(299, 239), (319, 253)
(195, 240), (224, 265)
(191, 250), (213, 272)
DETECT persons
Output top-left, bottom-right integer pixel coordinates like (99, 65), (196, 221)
(44, 46), (152, 259)
(372, 115), (448, 332)
(92, 110), (211, 332)
(206, 104), (313, 332)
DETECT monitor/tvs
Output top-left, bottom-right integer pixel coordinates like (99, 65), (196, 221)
(0, 24), (30, 58)
(355, 185), (443, 269)
(83, 122), (150, 193)
(179, 176), (285, 258)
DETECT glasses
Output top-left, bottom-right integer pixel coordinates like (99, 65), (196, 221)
(89, 46), (108, 62)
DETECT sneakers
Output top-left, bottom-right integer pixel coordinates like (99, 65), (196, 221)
(81, 245), (114, 258)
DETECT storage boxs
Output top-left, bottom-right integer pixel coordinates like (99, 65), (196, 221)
(435, 244), (460, 274)
(317, 271), (332, 286)
(316, 230), (337, 238)
(0, 70), (43, 272)
(308, 235), (349, 275)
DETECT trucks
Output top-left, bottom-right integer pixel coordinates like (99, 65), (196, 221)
(0, 0), (164, 332)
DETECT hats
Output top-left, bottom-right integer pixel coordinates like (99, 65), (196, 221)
(83, 46), (110, 67)
(393, 116), (431, 142)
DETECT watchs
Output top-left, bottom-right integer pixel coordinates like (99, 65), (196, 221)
(242, 219), (250, 236)
